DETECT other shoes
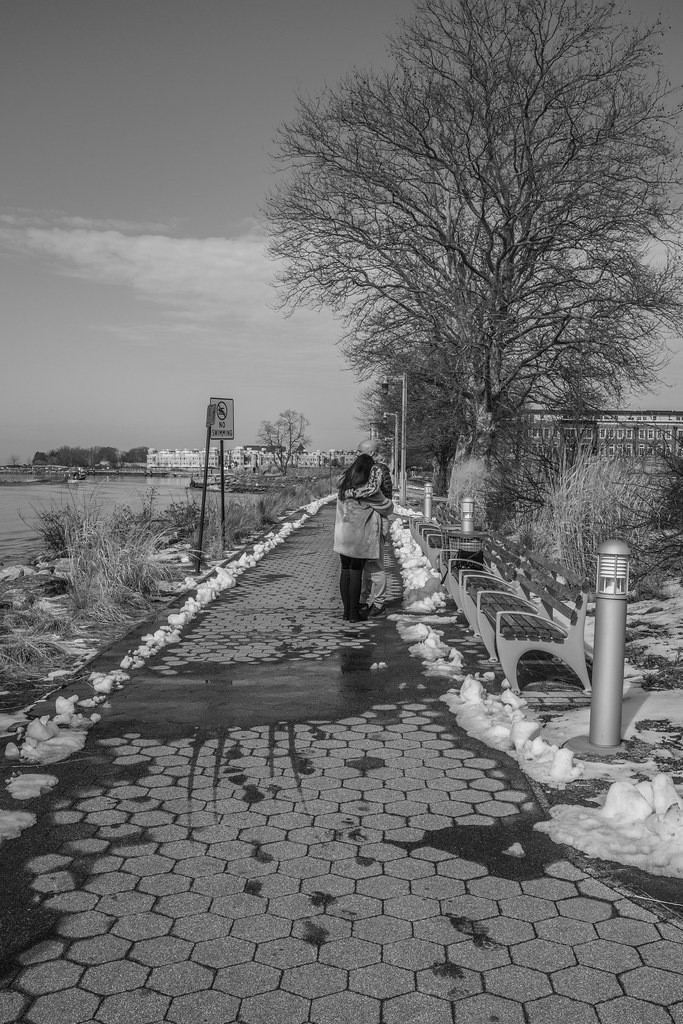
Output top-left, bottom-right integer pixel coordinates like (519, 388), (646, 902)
(367, 604), (387, 619)
(359, 602), (369, 614)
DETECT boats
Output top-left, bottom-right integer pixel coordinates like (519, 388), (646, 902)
(0, 462), (86, 486)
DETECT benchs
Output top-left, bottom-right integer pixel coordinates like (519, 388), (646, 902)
(409, 499), (593, 696)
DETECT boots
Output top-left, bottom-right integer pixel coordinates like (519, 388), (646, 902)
(340, 569), (368, 623)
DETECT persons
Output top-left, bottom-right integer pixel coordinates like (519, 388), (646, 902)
(333, 454), (395, 623)
(337, 438), (392, 620)
